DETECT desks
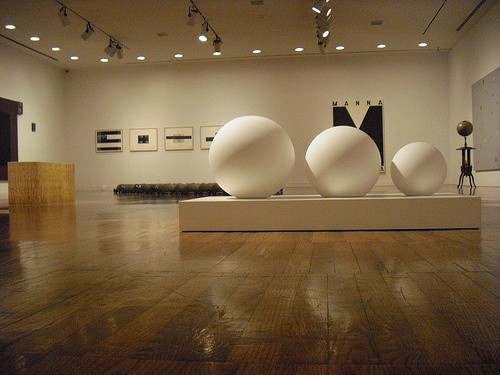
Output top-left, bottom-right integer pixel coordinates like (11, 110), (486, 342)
(8, 161), (77, 205)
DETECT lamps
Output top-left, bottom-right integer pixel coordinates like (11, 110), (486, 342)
(80, 22), (94, 41)
(198, 22), (209, 41)
(313, 0), (332, 48)
(58, 6), (71, 26)
(212, 34), (221, 56)
(186, 7), (198, 26)
(104, 39), (124, 59)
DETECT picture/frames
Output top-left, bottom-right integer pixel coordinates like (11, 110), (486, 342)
(200, 125), (224, 150)
(96, 128), (123, 153)
(164, 126), (194, 150)
(129, 128), (158, 151)
